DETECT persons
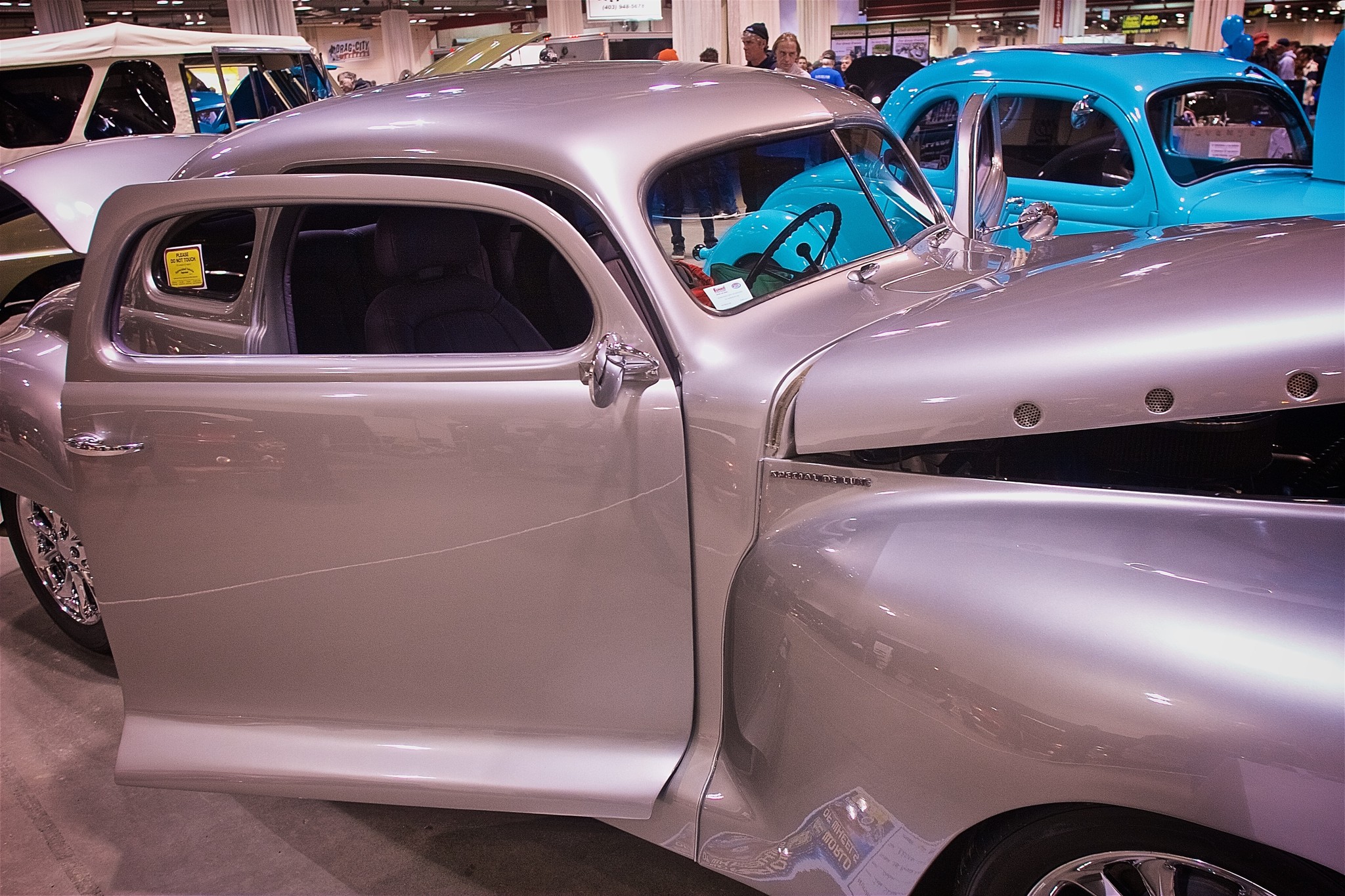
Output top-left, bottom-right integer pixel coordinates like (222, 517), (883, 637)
(1249, 31), (1330, 116)
(337, 71), (377, 95)
(654, 22), (855, 259)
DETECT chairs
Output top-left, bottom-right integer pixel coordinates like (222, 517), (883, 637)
(1036, 131), (1119, 185)
(365, 206), (552, 351)
(515, 226), (589, 342)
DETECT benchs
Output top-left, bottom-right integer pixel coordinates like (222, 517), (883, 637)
(230, 214), (517, 355)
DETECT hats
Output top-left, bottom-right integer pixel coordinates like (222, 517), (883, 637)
(823, 51), (834, 60)
(1277, 38), (1290, 47)
(1253, 32), (1270, 45)
(745, 22), (768, 45)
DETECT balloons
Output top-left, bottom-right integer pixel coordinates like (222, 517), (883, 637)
(1231, 35), (1255, 61)
(1220, 14), (1245, 46)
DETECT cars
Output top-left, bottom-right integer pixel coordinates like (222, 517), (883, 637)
(693, 16), (1343, 277)
(0, 21), (553, 312)
(0, 57), (1343, 894)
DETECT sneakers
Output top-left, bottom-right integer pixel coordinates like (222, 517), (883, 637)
(714, 209), (741, 220)
(706, 242), (717, 250)
(671, 245), (685, 260)
